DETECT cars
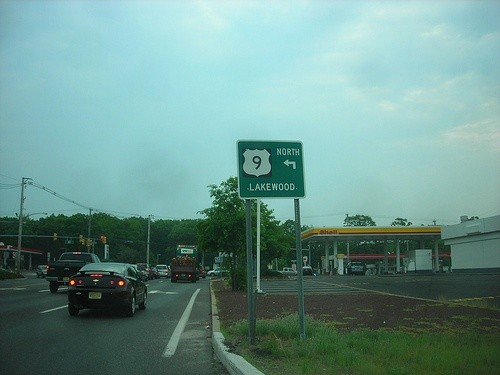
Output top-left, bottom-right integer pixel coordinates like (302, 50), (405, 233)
(67, 263), (148, 318)
(46, 252), (102, 294)
(302, 266), (314, 276)
(346, 261), (365, 274)
(130, 262), (160, 283)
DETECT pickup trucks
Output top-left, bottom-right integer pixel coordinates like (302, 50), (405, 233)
(277, 267), (297, 277)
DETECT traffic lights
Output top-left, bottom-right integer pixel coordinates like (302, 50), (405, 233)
(80, 235), (93, 247)
(103, 237), (107, 245)
(53, 233), (58, 241)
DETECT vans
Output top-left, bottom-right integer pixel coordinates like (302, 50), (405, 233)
(155, 265), (171, 278)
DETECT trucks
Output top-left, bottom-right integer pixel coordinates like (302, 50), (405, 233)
(170, 263), (197, 283)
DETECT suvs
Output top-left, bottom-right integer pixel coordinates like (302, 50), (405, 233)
(198, 265), (207, 278)
(206, 267), (230, 277)
(36, 265), (48, 278)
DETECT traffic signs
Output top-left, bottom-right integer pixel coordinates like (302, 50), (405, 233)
(237, 139), (304, 199)
